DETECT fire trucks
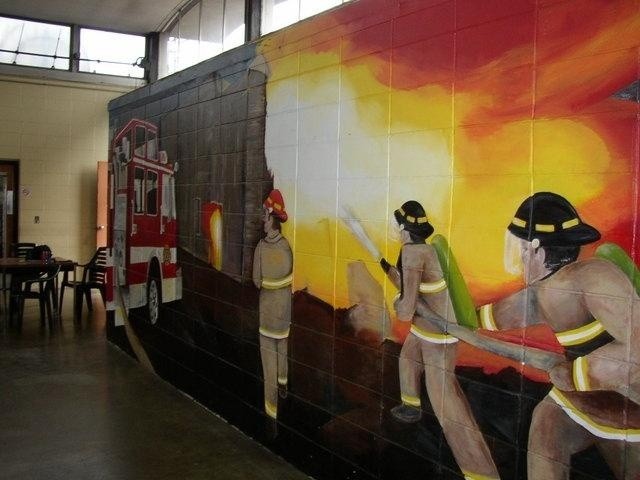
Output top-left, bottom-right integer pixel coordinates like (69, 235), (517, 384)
(107, 118), (184, 324)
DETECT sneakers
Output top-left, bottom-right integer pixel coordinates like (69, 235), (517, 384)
(392, 403), (421, 422)
(278, 382), (287, 397)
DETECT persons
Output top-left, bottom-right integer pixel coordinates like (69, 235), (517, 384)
(472, 190), (640, 480)
(251, 186), (293, 419)
(390, 199), (501, 480)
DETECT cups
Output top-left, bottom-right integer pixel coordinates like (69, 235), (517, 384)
(41, 251), (51, 264)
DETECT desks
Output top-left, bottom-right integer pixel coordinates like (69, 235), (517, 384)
(0, 258), (75, 327)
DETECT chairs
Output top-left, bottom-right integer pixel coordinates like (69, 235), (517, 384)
(7, 242), (44, 314)
(59, 246), (112, 316)
(8, 261), (62, 330)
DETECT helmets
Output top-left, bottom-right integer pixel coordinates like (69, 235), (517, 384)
(508, 192), (601, 244)
(394, 201), (434, 235)
(263, 190), (288, 222)
